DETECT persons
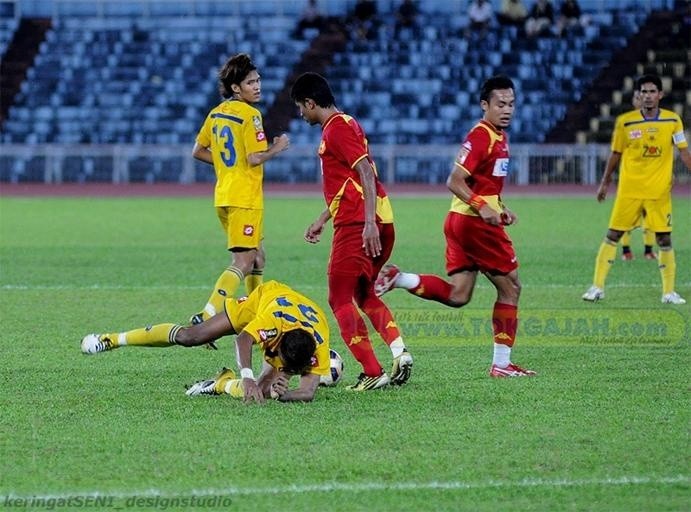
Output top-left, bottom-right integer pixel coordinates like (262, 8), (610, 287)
(290, 72), (414, 390)
(555, 0), (582, 36)
(526, 0), (554, 35)
(188, 52), (291, 351)
(583, 78), (691, 305)
(493, 0), (527, 37)
(80, 280), (329, 406)
(374, 77), (538, 377)
(394, 0), (422, 41)
(290, 0), (344, 42)
(345, 1), (386, 41)
(620, 84), (658, 260)
(468, 1), (494, 31)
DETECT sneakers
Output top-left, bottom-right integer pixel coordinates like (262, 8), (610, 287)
(82, 333), (113, 355)
(582, 287), (605, 302)
(489, 362), (538, 380)
(623, 252), (632, 259)
(645, 252), (653, 256)
(390, 352), (413, 386)
(185, 368), (235, 397)
(191, 311), (220, 353)
(344, 371), (389, 393)
(661, 292), (682, 305)
(374, 265), (400, 298)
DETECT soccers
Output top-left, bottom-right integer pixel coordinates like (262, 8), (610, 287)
(318, 349), (344, 387)
